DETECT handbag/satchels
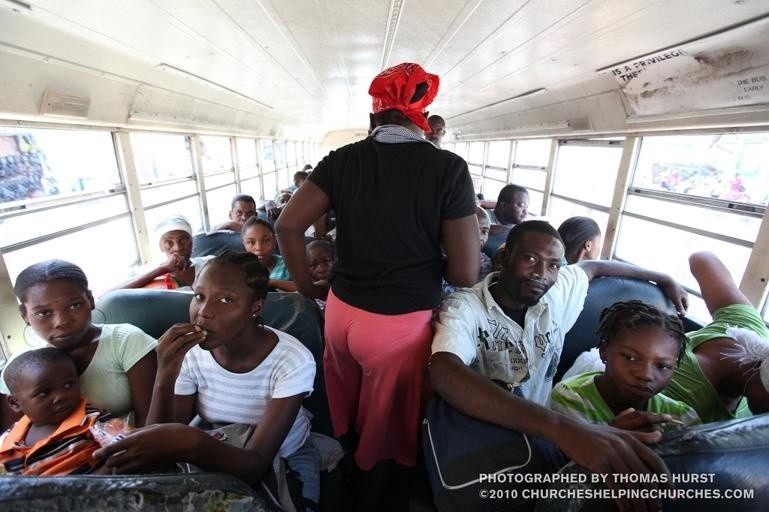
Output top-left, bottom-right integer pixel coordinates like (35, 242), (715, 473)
(175, 423), (308, 512)
(420, 378), (549, 511)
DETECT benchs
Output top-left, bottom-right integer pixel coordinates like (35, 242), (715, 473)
(533, 414), (765, 512)
(192, 233), (314, 257)
(255, 208), (285, 232)
(91, 287), (334, 437)
(552, 276), (681, 387)
(0, 472), (270, 512)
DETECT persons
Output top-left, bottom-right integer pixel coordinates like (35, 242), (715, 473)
(274, 63), (481, 511)
(1, 169), (769, 511)
(422, 114), (448, 150)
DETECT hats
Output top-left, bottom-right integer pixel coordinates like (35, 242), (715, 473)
(275, 190), (293, 204)
(156, 218), (193, 244)
